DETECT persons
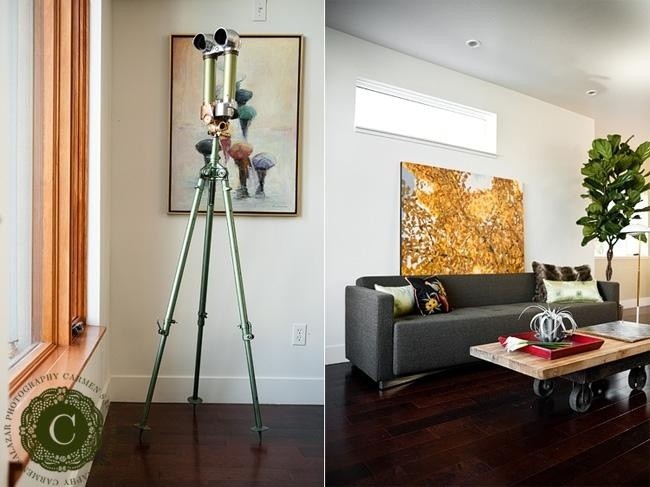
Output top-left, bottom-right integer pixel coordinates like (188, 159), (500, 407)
(256, 166), (268, 192)
(235, 156), (253, 187)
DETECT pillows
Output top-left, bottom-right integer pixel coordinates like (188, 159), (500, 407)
(374, 283), (415, 318)
(405, 274), (452, 317)
(543, 278), (605, 303)
(531, 265), (592, 303)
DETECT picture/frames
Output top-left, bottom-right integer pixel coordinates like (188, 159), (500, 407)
(165, 34), (303, 217)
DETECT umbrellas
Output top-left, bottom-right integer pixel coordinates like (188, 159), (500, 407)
(195, 89), (278, 168)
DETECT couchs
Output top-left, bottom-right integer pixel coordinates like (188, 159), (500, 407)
(346, 273), (623, 390)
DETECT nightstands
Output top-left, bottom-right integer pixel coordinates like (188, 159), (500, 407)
(470, 320), (650, 413)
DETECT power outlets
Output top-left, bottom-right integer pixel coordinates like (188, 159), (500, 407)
(292, 324), (306, 346)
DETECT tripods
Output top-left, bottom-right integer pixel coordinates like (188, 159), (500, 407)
(133, 138), (269, 445)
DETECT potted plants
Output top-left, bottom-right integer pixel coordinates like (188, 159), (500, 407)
(576, 134), (650, 319)
